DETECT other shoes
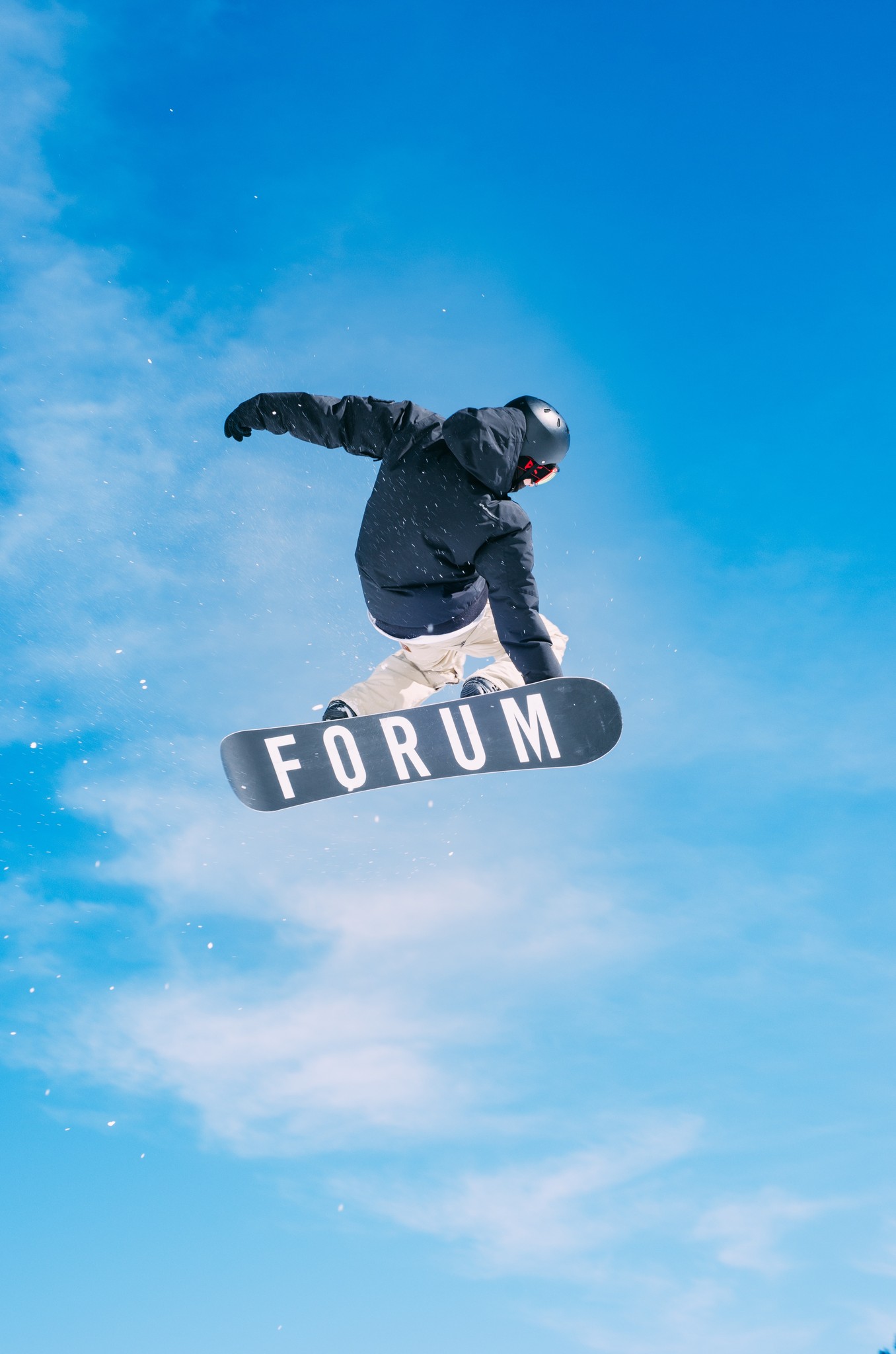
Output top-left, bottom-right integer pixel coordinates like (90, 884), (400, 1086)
(322, 700), (357, 722)
(459, 678), (500, 698)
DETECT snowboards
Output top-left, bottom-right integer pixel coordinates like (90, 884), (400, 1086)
(221, 674), (623, 813)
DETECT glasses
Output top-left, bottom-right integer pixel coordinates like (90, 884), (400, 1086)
(532, 467), (559, 487)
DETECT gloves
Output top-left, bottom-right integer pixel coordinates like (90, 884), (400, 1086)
(223, 404), (252, 442)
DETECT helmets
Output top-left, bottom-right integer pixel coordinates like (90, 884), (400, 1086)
(503, 395), (570, 476)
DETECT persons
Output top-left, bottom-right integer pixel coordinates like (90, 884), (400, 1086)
(224, 395), (571, 723)
